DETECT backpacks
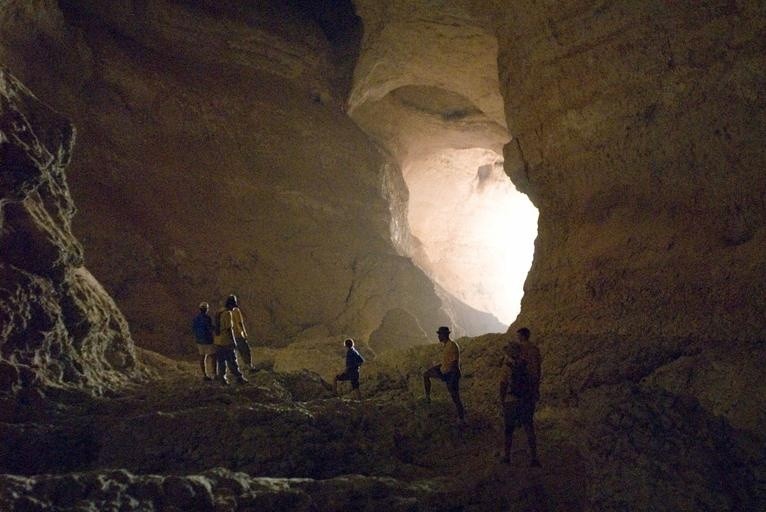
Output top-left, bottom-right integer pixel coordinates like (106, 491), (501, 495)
(505, 359), (532, 398)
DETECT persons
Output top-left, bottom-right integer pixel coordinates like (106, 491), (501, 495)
(231, 308), (260, 372)
(332, 338), (365, 400)
(191, 301), (220, 383)
(498, 340), (539, 468)
(420, 325), (466, 423)
(212, 294), (248, 385)
(515, 327), (542, 424)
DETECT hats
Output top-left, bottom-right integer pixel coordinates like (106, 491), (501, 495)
(226, 295), (239, 306)
(200, 302), (209, 308)
(436, 327), (452, 334)
